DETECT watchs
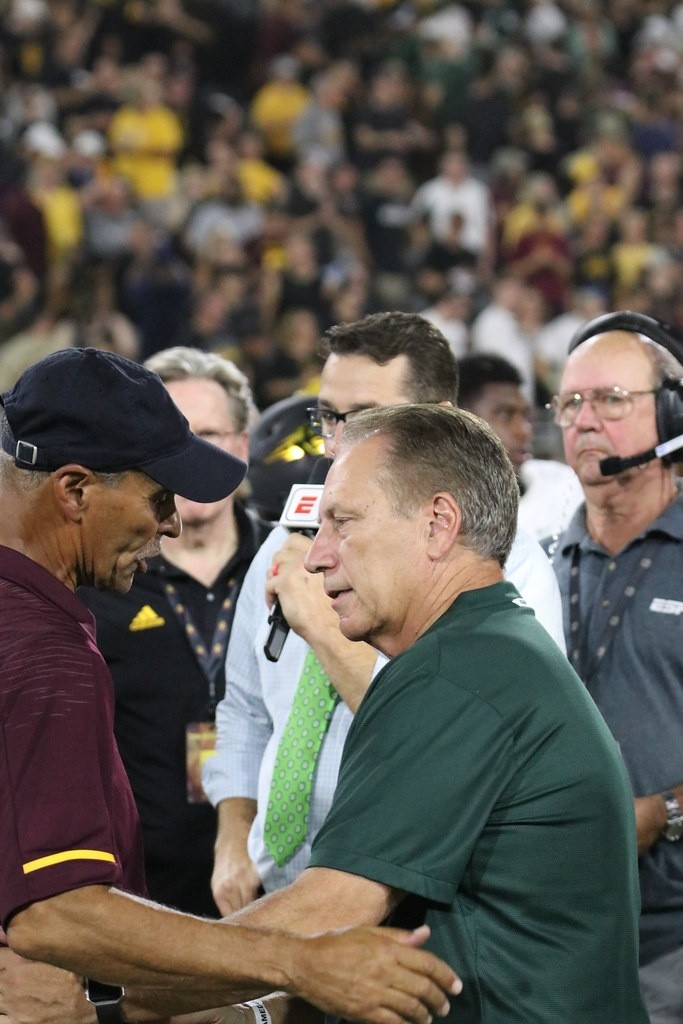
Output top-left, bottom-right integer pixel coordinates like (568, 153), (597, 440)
(85, 978), (125, 1024)
(660, 791), (683, 842)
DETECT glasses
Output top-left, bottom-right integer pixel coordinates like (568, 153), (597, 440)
(545, 387), (657, 428)
(305, 406), (363, 437)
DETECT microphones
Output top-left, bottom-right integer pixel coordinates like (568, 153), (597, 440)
(263, 458), (333, 662)
(599, 435), (683, 476)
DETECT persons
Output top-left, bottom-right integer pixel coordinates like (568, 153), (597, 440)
(0, 0), (683, 1024)
(0, 402), (653, 1024)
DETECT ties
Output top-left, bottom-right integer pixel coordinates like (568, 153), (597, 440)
(264, 647), (340, 867)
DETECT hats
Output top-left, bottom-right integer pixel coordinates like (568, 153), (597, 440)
(1, 348), (248, 503)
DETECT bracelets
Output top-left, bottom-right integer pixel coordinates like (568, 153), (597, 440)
(244, 999), (272, 1024)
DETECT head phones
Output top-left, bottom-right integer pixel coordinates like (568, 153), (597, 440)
(567, 308), (683, 468)
